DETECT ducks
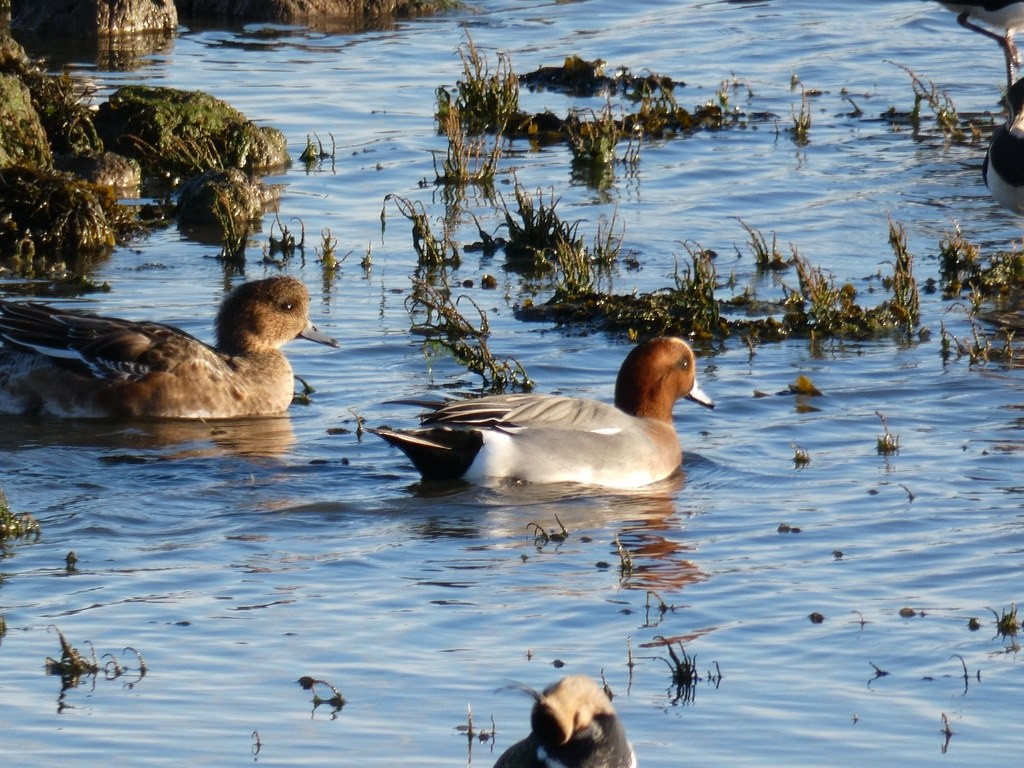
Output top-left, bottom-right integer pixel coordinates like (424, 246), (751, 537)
(981, 76), (1024, 218)
(489, 674), (638, 768)
(360, 334), (717, 492)
(0, 274), (342, 420)
(935, 0), (1024, 87)
